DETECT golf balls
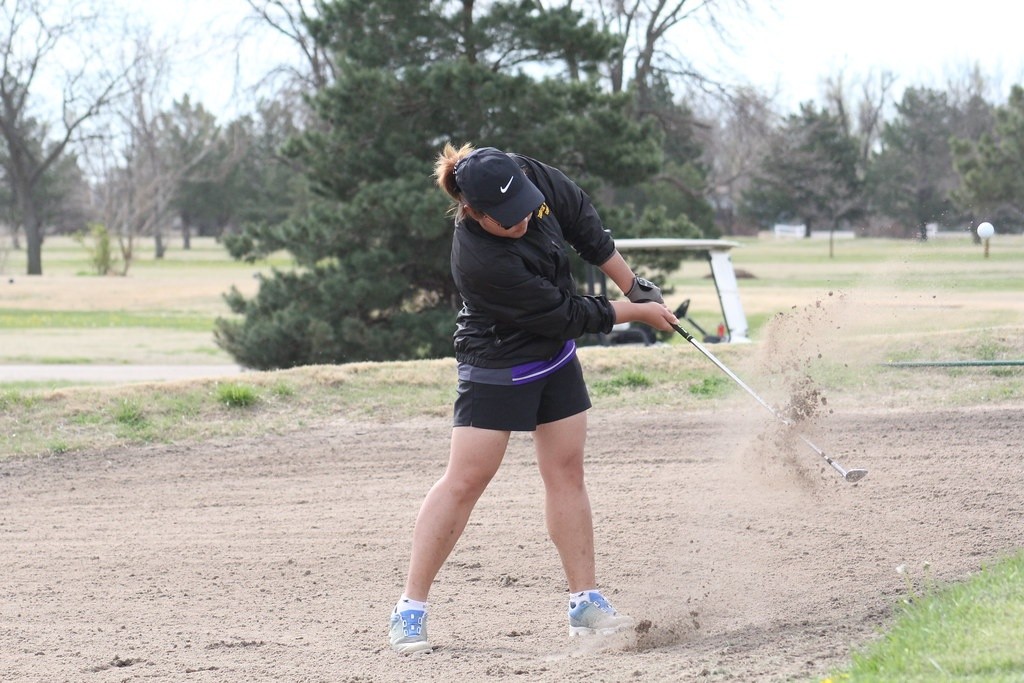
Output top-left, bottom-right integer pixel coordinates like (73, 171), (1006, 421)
(977, 221), (995, 239)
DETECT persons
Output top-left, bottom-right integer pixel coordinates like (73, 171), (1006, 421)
(389, 141), (679, 655)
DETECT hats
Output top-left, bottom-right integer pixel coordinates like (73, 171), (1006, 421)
(456, 147), (545, 227)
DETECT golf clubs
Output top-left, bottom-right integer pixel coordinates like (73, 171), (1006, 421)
(669, 322), (870, 483)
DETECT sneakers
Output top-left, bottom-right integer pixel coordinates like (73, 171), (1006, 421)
(568, 593), (634, 638)
(389, 609), (433, 655)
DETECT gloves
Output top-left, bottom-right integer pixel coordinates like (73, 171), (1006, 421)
(624, 276), (664, 303)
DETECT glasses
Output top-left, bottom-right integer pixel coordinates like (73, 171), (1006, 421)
(480, 211), (514, 230)
